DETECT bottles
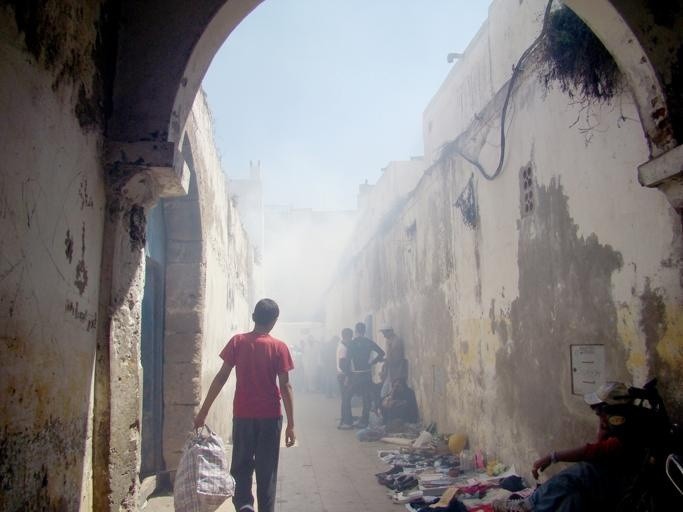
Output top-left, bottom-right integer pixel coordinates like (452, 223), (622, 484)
(459, 450), (476, 474)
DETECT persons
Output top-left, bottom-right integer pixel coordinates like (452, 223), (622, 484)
(194, 299), (294, 511)
(290, 323), (418, 435)
(491, 382), (656, 512)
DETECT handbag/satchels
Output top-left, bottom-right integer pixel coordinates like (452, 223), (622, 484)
(174, 424), (235, 512)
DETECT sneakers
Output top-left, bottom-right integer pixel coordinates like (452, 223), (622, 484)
(337, 420), (367, 430)
(491, 497), (528, 512)
(376, 463), (423, 502)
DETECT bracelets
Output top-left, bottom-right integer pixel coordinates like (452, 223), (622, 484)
(551, 450), (557, 465)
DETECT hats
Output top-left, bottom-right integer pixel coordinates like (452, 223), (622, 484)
(584, 381), (629, 405)
(377, 324), (392, 331)
